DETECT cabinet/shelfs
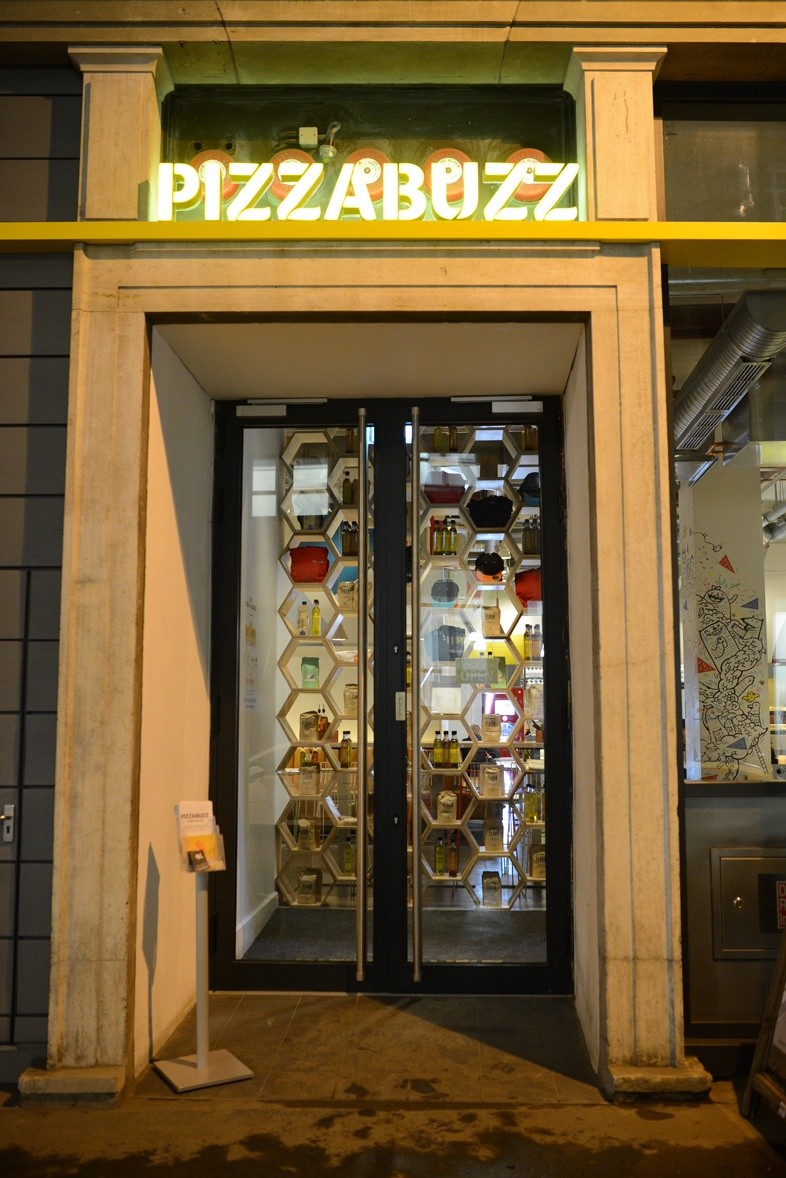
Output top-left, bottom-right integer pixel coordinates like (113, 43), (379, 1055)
(684, 796), (786, 1023)
(275, 425), (546, 910)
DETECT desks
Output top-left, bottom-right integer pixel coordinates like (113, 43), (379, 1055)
(331, 741), (545, 748)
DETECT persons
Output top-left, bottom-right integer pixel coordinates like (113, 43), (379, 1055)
(461, 725), (500, 778)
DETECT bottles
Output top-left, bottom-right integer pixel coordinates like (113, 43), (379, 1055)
(341, 731), (352, 768)
(432, 566), (459, 602)
(525, 425), (533, 451)
(299, 747), (320, 795)
(343, 837), (353, 876)
(524, 624), (532, 661)
(532, 518), (539, 554)
(311, 599), (321, 637)
(341, 521), (352, 556)
(476, 653), (486, 688)
(433, 520), (442, 555)
(441, 521), (450, 555)
(523, 519), (532, 554)
(449, 425), (457, 452)
(525, 784), (545, 824)
(351, 521), (359, 556)
(486, 652), (494, 688)
(345, 430), (354, 453)
(432, 731), (460, 768)
(434, 427), (442, 451)
(450, 520), (458, 555)
(532, 625), (541, 661)
(434, 837), (458, 878)
(298, 601), (309, 637)
(343, 472), (353, 505)
(317, 704), (327, 740)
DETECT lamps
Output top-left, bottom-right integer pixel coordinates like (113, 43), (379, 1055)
(280, 119), (342, 163)
